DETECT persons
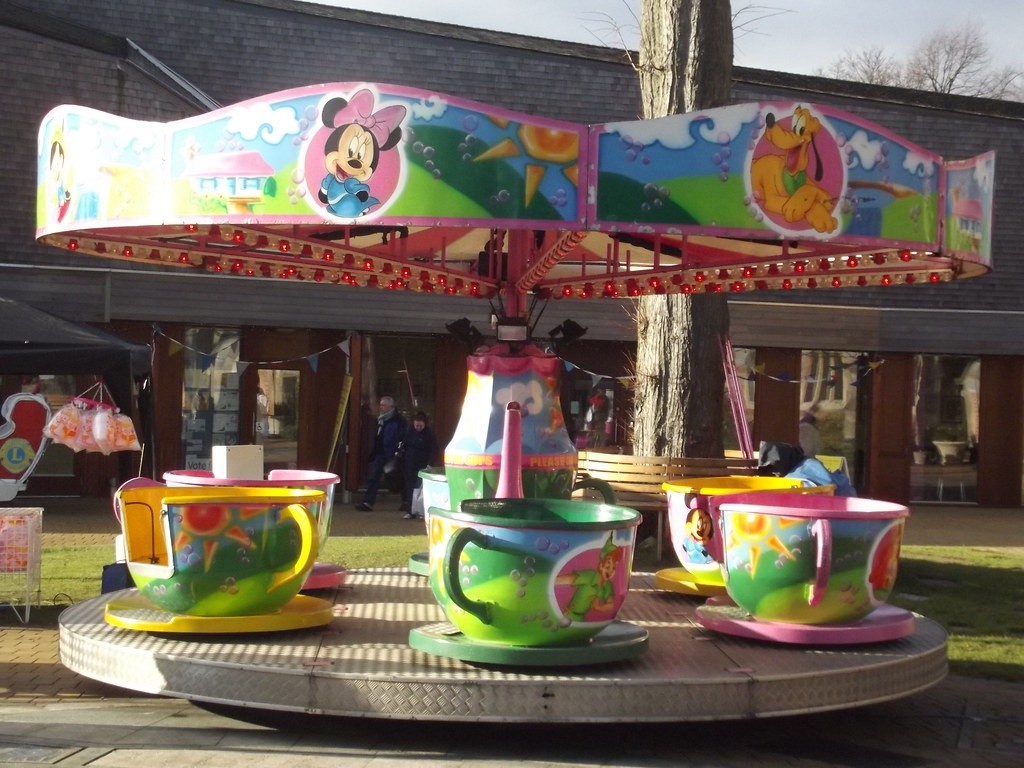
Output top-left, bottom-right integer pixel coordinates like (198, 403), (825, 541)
(356, 396), (407, 511)
(758, 440), (855, 496)
(74, 452), (118, 498)
(402, 412), (436, 519)
(800, 416), (818, 455)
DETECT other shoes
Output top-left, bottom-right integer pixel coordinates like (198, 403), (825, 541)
(353, 502), (373, 511)
(398, 501), (409, 511)
(402, 514), (412, 519)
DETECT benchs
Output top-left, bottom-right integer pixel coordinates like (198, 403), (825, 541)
(574, 445), (763, 568)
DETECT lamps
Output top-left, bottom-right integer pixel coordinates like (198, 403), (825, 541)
(547, 317), (590, 343)
(496, 317), (533, 358)
(445, 317), (487, 351)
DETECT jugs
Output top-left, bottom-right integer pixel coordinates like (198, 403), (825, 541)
(444, 343), (578, 516)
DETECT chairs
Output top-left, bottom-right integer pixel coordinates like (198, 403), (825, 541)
(930, 438), (968, 503)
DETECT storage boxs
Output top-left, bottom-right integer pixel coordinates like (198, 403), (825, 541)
(213, 413), (239, 433)
(183, 391), (209, 412)
(186, 439), (203, 451)
(0, 507), (44, 571)
(186, 418), (207, 433)
(186, 454), (210, 469)
(184, 369), (210, 387)
(213, 391), (241, 411)
(210, 445), (265, 481)
(211, 433), (239, 446)
(211, 369), (239, 389)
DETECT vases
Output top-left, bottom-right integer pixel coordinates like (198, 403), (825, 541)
(913, 451), (928, 465)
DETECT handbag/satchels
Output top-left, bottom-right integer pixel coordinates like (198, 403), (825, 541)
(412, 481), (424, 515)
(586, 407), (593, 422)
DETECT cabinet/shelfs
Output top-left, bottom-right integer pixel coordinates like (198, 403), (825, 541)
(184, 386), (241, 459)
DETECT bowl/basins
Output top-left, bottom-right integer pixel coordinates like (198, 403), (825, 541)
(914, 451), (930, 465)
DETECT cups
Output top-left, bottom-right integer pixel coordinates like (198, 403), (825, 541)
(426, 497), (644, 648)
(662, 476), (837, 586)
(417, 465), (617, 566)
(116, 486), (328, 618)
(162, 469), (340, 560)
(708, 488), (911, 627)
